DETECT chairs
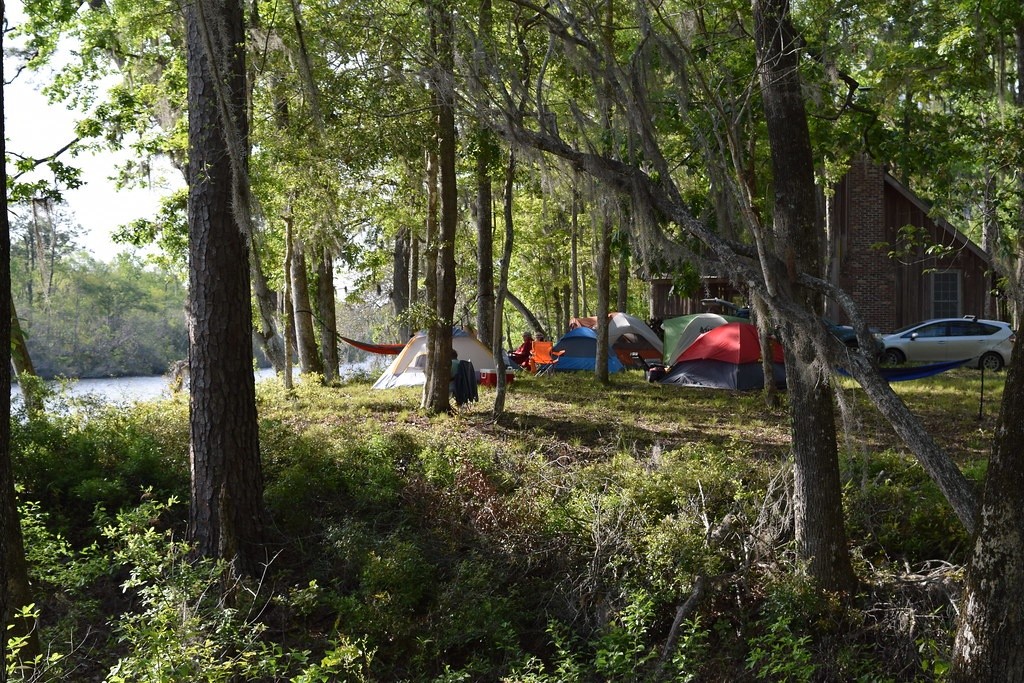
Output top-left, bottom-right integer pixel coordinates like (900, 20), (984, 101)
(528, 340), (565, 379)
(507, 348), (531, 373)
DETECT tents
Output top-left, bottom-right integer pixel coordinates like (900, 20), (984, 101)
(372, 325), (518, 390)
(540, 311), (864, 392)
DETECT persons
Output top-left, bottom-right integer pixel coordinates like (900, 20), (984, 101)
(509, 330), (546, 372)
(448, 349), (460, 397)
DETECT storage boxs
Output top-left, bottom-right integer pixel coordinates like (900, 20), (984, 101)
(630, 353), (667, 382)
(479, 369), (515, 388)
